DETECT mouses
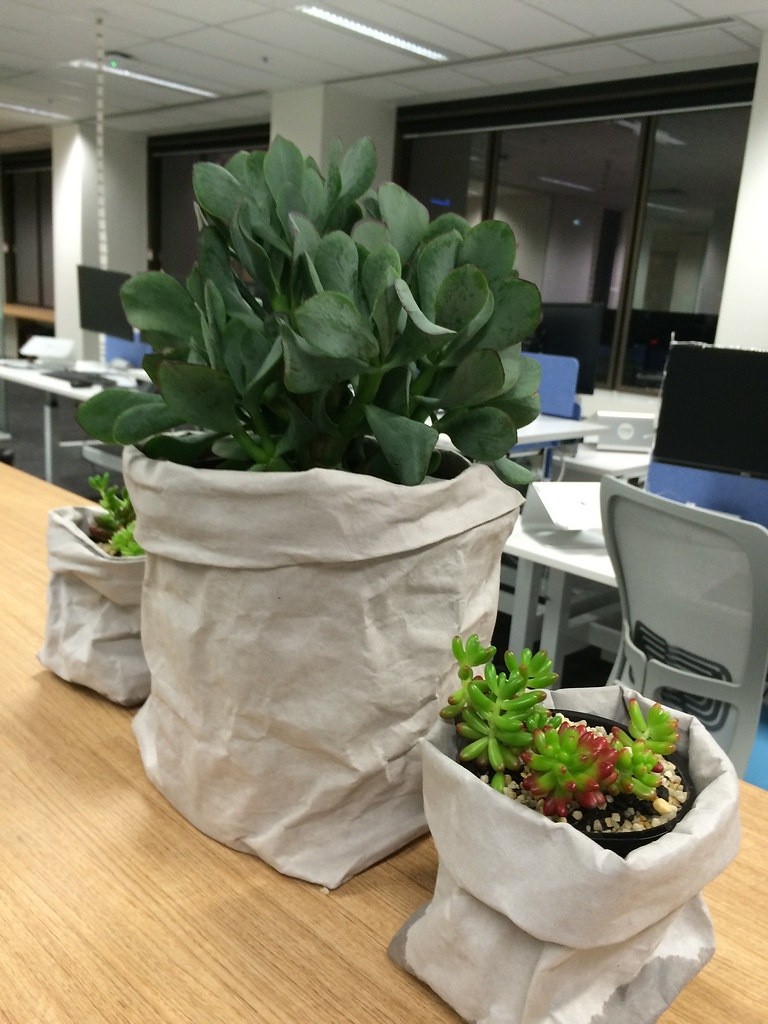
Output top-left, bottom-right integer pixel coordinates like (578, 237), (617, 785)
(71, 379), (92, 387)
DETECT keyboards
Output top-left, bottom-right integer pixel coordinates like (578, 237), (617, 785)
(43, 369), (102, 383)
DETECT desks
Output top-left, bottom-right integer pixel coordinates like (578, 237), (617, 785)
(1, 357), (153, 482)
(418, 405), (607, 670)
(3, 463), (765, 1024)
(504, 478), (625, 690)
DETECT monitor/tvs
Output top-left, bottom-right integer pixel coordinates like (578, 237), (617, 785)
(77, 265), (134, 342)
(650, 342), (768, 480)
(521, 302), (606, 395)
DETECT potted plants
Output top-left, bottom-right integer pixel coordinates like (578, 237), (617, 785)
(43, 141), (744, 1024)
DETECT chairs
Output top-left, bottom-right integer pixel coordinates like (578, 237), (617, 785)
(0, 334), (768, 782)
